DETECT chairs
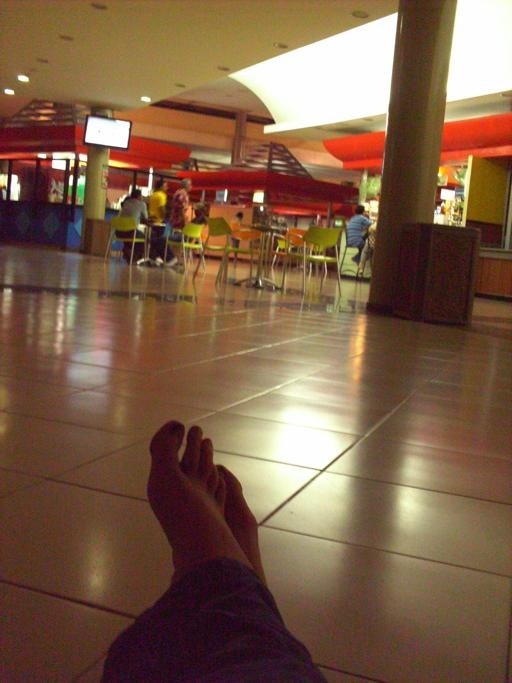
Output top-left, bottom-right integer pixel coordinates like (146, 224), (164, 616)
(103, 204), (372, 300)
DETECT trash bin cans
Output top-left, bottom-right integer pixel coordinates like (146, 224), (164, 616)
(84, 218), (112, 257)
(392, 222), (482, 327)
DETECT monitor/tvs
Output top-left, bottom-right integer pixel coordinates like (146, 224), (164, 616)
(83, 114), (132, 151)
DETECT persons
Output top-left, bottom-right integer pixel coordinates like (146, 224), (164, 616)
(99, 419), (326, 682)
(113, 179), (193, 268)
(230, 212), (243, 248)
(345, 205), (373, 264)
